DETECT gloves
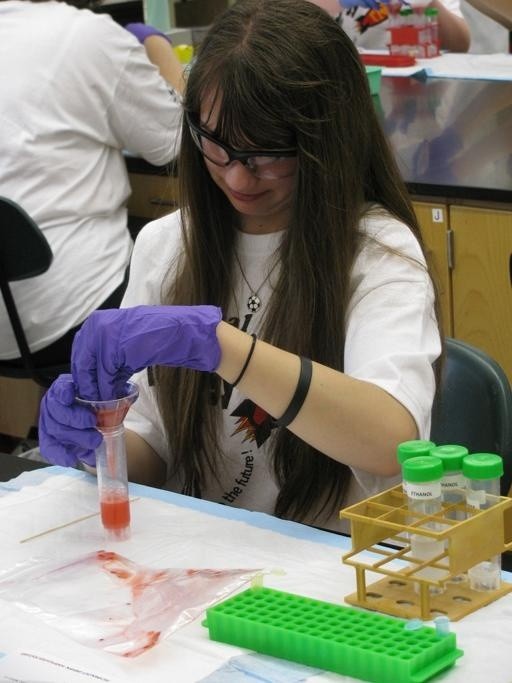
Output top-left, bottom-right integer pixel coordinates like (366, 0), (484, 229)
(38, 373), (103, 471)
(71, 304), (222, 408)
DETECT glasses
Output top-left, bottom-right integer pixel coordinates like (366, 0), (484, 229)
(185, 112), (300, 180)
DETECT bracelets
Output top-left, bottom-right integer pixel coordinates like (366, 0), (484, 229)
(271, 355), (314, 428)
(230, 333), (258, 389)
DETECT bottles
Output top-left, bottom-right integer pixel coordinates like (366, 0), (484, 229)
(384, 0), (440, 54)
(393, 436), (505, 598)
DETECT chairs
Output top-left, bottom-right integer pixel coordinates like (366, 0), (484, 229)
(431, 337), (512, 571)
(0, 195), (52, 454)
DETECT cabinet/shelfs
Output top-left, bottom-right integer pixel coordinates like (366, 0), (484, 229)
(125, 172), (511, 390)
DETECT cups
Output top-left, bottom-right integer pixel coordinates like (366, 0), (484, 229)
(94, 423), (134, 542)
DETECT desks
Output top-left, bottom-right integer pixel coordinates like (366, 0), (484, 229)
(0, 453), (512, 681)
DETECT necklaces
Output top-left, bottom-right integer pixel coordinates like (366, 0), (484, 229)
(231, 241), (284, 312)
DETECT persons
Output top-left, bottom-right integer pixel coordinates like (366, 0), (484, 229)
(306, 0), (472, 52)
(0, 0), (185, 391)
(36, 0), (446, 550)
